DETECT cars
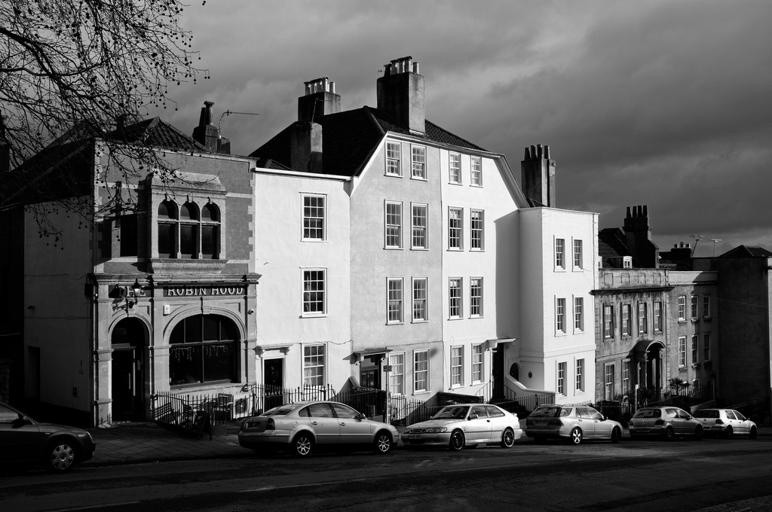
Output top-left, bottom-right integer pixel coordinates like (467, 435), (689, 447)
(629, 405), (705, 441)
(525, 404), (624, 445)
(0, 401), (96, 473)
(693, 408), (758, 439)
(401, 403), (523, 453)
(238, 400), (401, 458)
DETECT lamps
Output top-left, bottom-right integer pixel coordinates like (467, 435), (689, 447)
(128, 277), (142, 307)
